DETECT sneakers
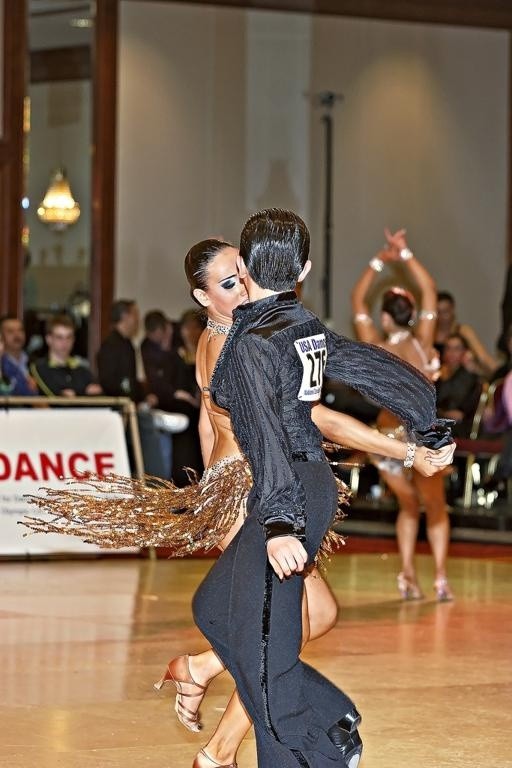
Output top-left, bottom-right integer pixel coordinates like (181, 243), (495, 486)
(152, 410), (189, 434)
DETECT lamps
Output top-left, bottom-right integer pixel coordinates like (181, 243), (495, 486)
(36, 108), (82, 233)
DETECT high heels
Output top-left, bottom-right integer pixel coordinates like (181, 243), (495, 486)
(153, 654), (206, 732)
(193, 747), (237, 768)
(433, 578), (454, 601)
(396, 573), (423, 599)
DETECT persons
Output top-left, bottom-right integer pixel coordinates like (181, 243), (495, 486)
(1, 246), (510, 566)
(188, 205), (457, 767)
(14, 239), (449, 768)
(349, 227), (459, 603)
